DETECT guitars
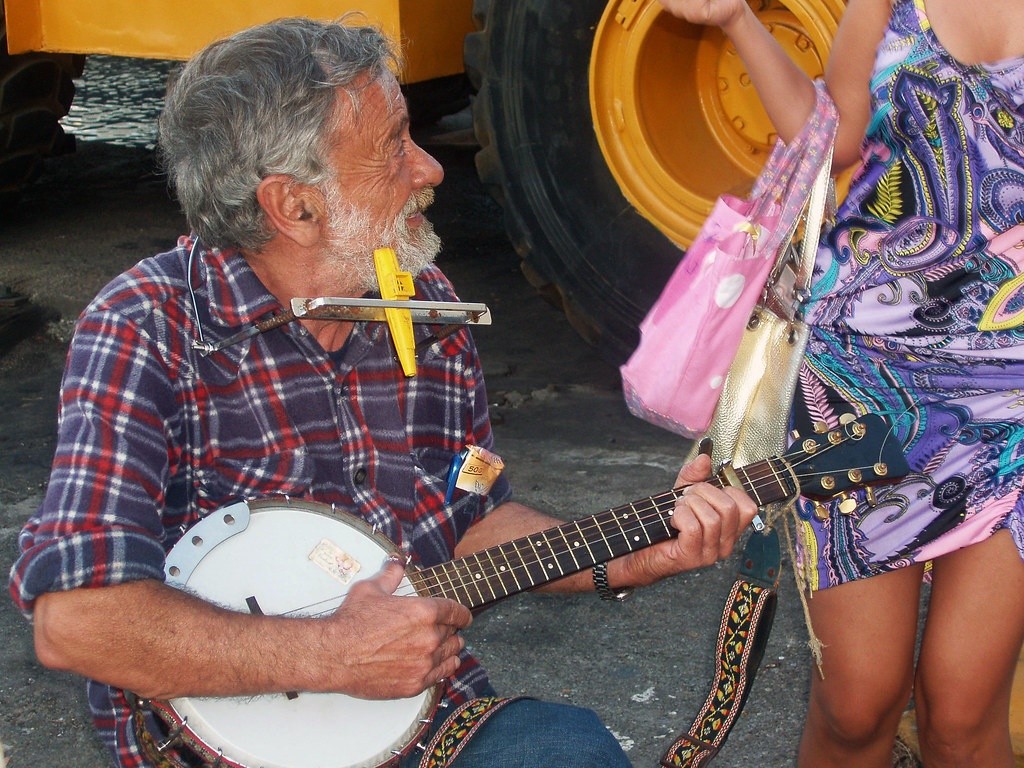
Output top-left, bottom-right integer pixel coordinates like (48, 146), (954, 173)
(125, 402), (912, 768)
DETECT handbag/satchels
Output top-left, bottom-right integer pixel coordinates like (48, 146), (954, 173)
(620, 79), (839, 441)
(685, 144), (834, 524)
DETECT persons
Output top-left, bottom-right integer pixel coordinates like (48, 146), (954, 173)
(658, 1), (1024, 768)
(6, 10), (757, 768)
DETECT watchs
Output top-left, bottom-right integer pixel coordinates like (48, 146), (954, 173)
(593, 562), (635, 601)
